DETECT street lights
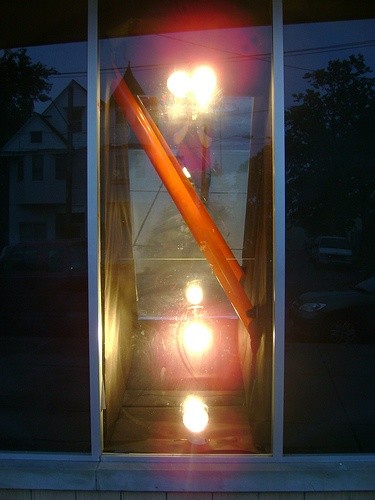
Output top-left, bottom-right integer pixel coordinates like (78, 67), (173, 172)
(39, 86), (74, 234)
(167, 62), (219, 211)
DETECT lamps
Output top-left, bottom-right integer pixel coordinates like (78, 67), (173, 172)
(177, 279), (213, 436)
(166, 64), (220, 108)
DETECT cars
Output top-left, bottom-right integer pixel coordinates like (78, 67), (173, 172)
(292, 270), (375, 345)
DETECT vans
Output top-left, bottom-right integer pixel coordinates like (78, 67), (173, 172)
(0, 236), (88, 336)
(310, 237), (353, 269)
